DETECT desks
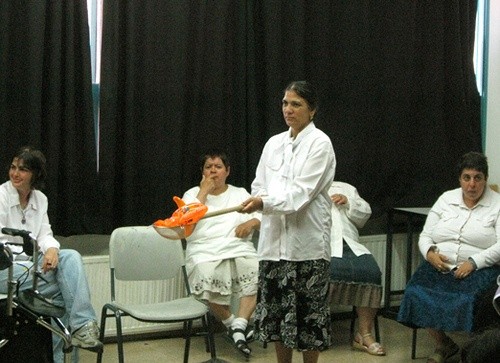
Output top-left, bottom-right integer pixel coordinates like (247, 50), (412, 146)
(384, 207), (431, 314)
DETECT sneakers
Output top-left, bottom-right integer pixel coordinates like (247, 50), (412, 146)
(71, 320), (103, 352)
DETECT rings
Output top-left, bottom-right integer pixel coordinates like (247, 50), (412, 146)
(438, 264), (441, 268)
(339, 194), (343, 198)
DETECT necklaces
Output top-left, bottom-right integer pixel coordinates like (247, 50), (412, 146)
(20, 209), (27, 224)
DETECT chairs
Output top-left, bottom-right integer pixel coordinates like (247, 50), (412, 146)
(96, 226), (500, 363)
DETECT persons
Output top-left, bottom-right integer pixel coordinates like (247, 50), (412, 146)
(459, 328), (500, 363)
(398, 151), (500, 363)
(0, 146), (103, 363)
(181, 150), (262, 358)
(325, 181), (386, 355)
(238, 81), (337, 363)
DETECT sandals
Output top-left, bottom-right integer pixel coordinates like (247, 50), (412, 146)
(245, 325), (255, 343)
(222, 325), (251, 357)
(427, 337), (458, 363)
(353, 333), (385, 355)
